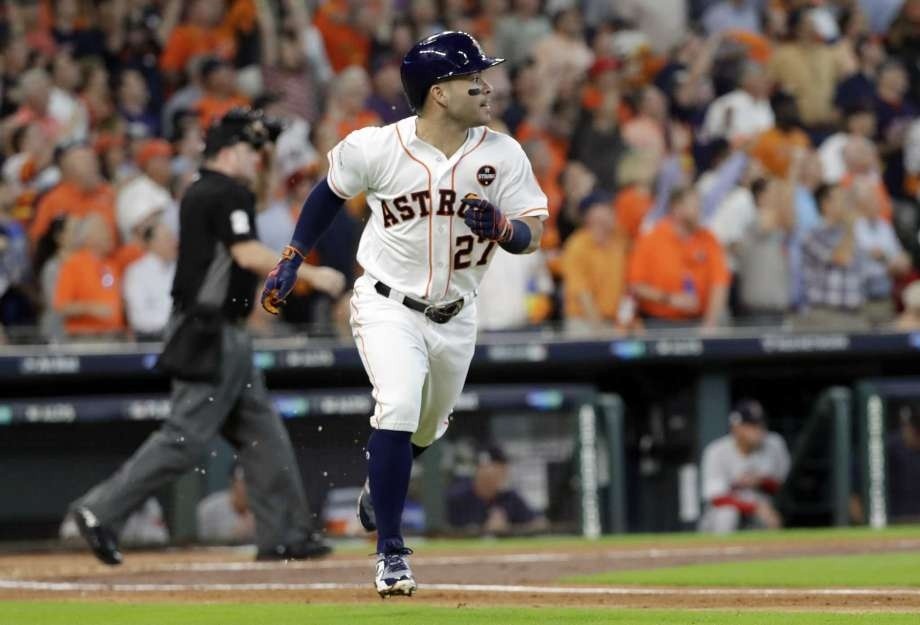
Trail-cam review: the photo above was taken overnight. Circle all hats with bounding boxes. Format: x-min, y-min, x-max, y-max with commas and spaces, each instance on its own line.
736, 401, 766, 422
137, 138, 176, 166
201, 120, 252, 152
580, 189, 614, 211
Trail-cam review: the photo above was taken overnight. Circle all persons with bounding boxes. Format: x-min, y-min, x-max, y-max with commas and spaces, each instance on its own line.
258, 31, 549, 595
346, 465, 426, 540
848, 404, 920, 525
0, 0, 920, 338
66, 101, 345, 566
696, 401, 792, 533
442, 447, 551, 539
62, 464, 254, 548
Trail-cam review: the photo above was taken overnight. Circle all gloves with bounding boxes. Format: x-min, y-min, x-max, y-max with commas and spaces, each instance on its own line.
260, 246, 307, 316
461, 196, 511, 244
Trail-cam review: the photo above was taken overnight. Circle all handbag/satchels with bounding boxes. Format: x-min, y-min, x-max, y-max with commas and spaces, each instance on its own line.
151, 312, 221, 387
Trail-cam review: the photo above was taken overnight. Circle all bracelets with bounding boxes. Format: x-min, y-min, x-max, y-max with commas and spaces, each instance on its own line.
660, 290, 672, 306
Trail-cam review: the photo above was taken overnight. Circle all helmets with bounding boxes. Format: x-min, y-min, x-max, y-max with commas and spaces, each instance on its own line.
401, 31, 505, 114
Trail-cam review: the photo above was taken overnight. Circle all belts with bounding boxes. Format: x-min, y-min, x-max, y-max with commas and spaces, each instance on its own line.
375, 280, 464, 325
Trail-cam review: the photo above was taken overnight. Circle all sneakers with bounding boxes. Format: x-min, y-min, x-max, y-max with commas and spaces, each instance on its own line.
357, 476, 377, 532
254, 539, 332, 563
368, 547, 418, 600
67, 498, 123, 566
